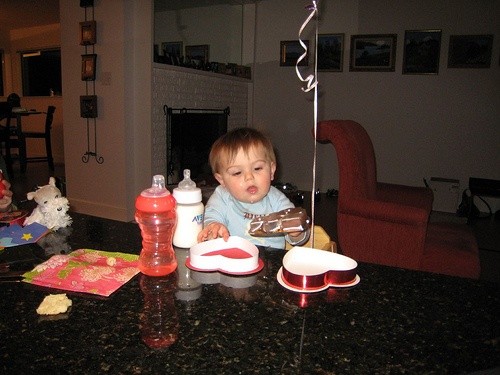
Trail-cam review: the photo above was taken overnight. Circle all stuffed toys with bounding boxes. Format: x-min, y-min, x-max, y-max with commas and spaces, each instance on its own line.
24, 176, 72, 230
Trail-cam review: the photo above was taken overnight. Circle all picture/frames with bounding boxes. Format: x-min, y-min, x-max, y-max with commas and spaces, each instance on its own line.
349, 33, 398, 72
160, 41, 183, 57
79, 95, 98, 118
314, 33, 345, 72
80, 54, 97, 81
401, 29, 442, 75
280, 40, 310, 67
447, 34, 494, 69
79, 20, 97, 46
185, 45, 210, 65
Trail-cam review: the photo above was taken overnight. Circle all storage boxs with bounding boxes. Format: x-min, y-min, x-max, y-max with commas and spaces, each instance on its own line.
429, 177, 460, 213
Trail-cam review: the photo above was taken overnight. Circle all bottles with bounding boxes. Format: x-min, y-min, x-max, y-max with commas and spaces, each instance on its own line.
171, 169, 205, 248
135, 175, 177, 276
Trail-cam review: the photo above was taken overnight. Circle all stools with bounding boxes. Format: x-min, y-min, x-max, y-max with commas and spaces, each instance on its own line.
467, 177, 500, 227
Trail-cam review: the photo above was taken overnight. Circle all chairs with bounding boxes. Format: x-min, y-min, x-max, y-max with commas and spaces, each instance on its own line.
16, 106, 56, 177
312, 119, 481, 279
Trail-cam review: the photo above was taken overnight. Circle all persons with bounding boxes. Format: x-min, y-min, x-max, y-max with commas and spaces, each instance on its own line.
0, 170, 17, 212
197, 127, 310, 250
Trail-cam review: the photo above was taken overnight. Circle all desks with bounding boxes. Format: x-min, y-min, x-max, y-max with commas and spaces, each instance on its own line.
0, 111, 48, 190
0, 211, 500, 375
